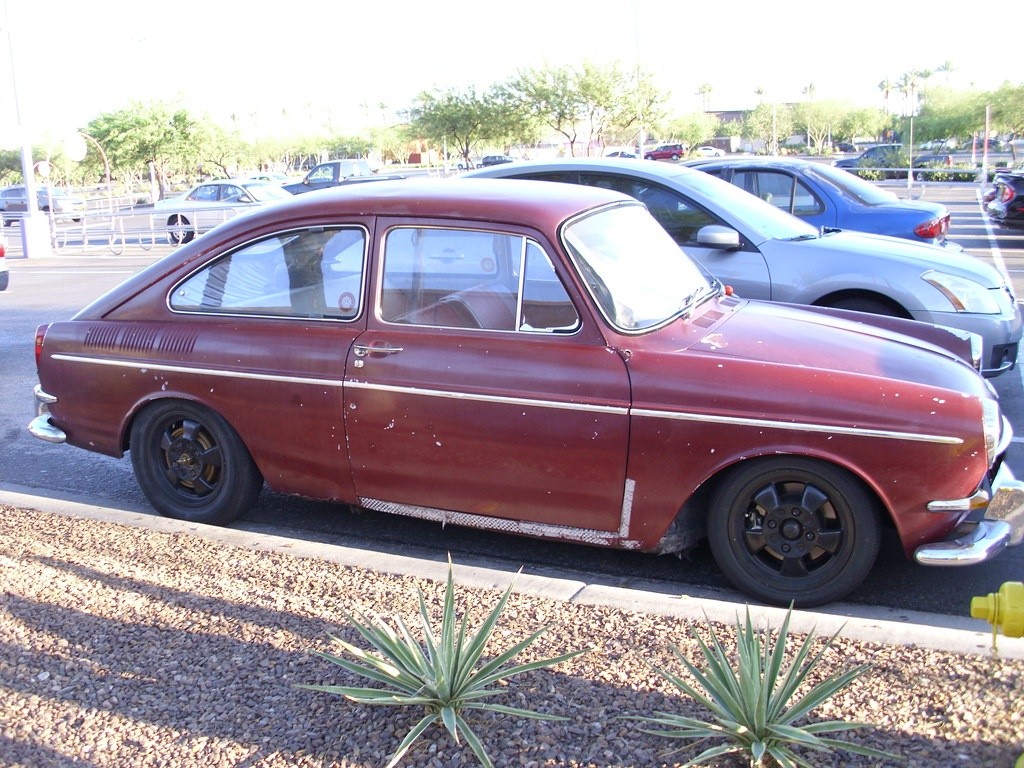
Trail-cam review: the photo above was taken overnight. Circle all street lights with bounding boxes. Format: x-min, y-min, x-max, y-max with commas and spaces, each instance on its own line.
64, 132, 114, 230
34, 161, 59, 250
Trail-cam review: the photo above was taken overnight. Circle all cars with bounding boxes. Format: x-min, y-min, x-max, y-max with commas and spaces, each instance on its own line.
482, 155, 514, 167
606, 151, 635, 158
27, 177, 1024, 608
693, 146, 726, 157
153, 178, 295, 243
460, 157, 1024, 380
831, 138, 1023, 230
689, 160, 964, 253
0, 186, 85, 226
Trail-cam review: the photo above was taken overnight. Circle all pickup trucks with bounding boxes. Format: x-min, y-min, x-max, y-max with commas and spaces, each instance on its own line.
281, 159, 407, 194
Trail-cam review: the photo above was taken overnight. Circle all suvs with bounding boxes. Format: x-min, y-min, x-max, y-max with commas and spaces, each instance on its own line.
644, 144, 684, 161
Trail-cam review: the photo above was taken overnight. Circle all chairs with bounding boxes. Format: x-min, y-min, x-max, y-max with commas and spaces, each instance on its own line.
391, 294, 485, 329
440, 281, 526, 332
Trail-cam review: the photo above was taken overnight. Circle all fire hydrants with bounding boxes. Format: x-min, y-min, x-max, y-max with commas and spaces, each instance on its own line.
970, 582, 1024, 768
